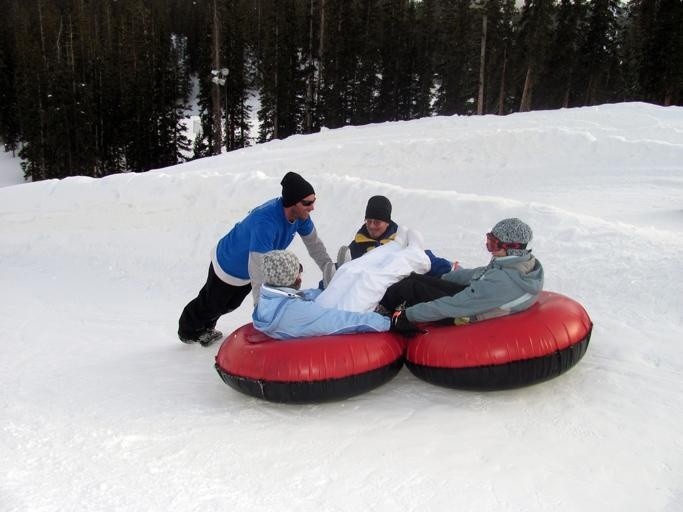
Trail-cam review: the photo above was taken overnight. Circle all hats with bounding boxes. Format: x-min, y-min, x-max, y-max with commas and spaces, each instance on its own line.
492, 218, 532, 244
365, 196, 391, 222
262, 250, 299, 286
281, 172, 314, 207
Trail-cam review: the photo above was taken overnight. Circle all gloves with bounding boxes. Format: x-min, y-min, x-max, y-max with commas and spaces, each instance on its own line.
425, 250, 451, 279
392, 310, 426, 338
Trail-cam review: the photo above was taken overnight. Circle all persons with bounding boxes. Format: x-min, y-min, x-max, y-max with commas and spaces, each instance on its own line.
253, 213, 440, 336
346, 196, 405, 257
323, 217, 545, 327
178, 171, 331, 343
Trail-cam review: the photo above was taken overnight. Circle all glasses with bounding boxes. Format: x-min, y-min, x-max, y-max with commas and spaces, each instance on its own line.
301, 200, 315, 206
486, 233, 526, 251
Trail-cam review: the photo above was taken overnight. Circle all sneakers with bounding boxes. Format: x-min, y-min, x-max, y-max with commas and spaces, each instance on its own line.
179, 329, 222, 346
